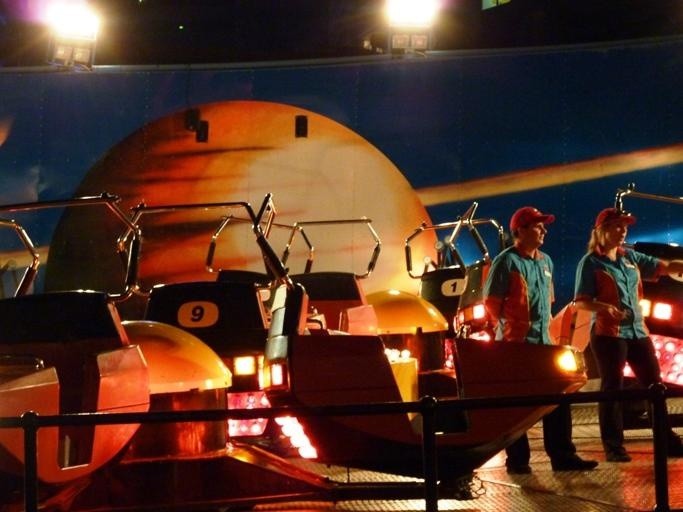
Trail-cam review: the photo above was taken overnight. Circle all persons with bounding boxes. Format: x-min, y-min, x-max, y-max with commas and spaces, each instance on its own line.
574, 207, 681, 462
483, 207, 598, 474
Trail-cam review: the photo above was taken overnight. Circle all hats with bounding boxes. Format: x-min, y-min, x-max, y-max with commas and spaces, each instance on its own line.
509, 205, 556, 232
593, 207, 637, 228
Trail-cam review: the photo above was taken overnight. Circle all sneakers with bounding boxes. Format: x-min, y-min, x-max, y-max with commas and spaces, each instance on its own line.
506, 462, 532, 474
666, 437, 682, 456
551, 455, 599, 471
605, 449, 631, 462
